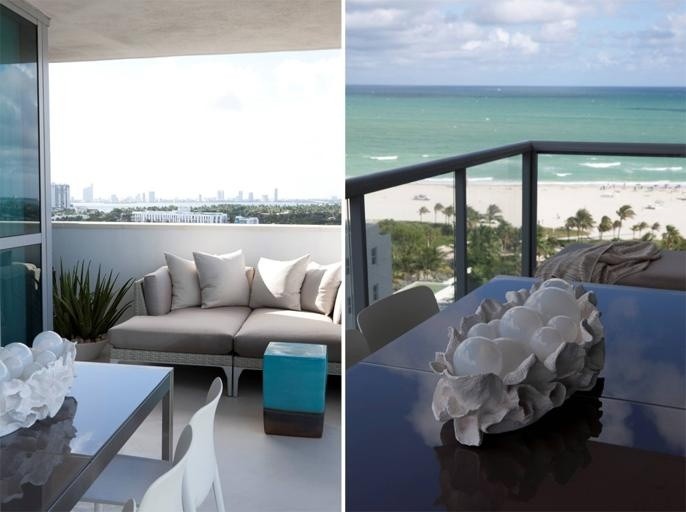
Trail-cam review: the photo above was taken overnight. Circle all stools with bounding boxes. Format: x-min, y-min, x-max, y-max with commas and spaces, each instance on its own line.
263, 340, 328, 437
539, 241, 685, 291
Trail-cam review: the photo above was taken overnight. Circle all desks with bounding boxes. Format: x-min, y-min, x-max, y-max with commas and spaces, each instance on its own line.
359, 272, 686, 410
345, 360, 686, 511
0, 361, 174, 512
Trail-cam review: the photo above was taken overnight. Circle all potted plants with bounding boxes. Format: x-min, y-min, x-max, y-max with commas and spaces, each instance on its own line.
52, 255, 136, 361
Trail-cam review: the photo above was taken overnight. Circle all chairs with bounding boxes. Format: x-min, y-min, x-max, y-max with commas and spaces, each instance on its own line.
345, 329, 371, 368
137, 425, 193, 512
79, 377, 224, 512
357, 284, 439, 355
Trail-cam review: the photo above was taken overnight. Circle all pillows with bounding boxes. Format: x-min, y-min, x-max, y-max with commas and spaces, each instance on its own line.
144, 250, 340, 315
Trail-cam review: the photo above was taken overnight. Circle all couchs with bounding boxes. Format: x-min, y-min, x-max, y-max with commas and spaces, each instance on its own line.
233, 277, 341, 398
107, 265, 253, 399
